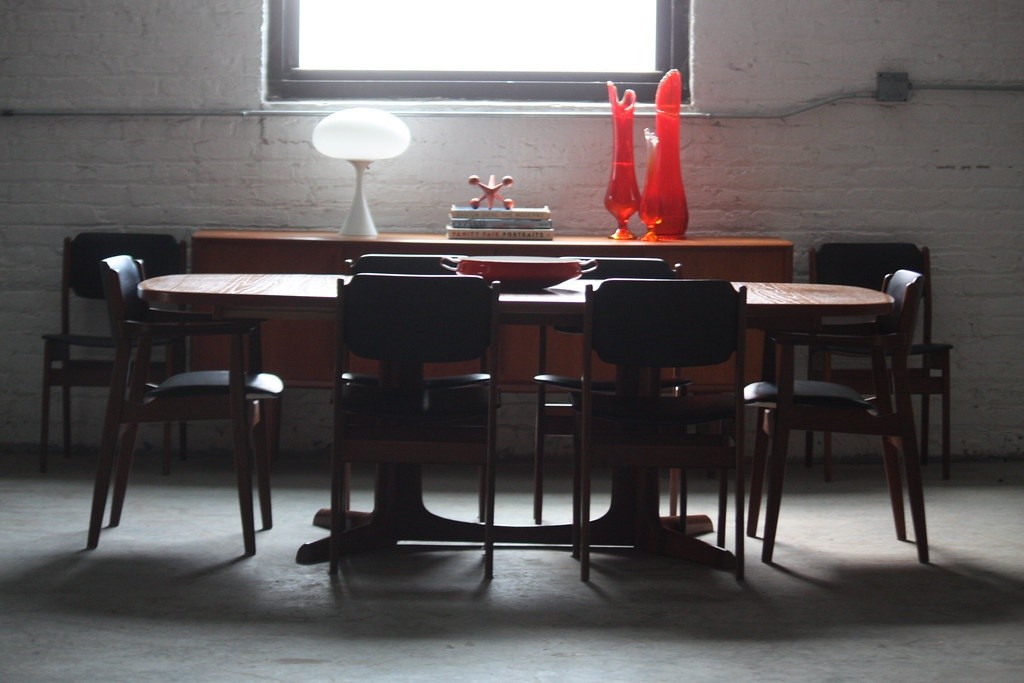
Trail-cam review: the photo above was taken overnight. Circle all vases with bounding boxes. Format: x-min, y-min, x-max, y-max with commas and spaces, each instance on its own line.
604, 80, 641, 240
638, 68, 690, 239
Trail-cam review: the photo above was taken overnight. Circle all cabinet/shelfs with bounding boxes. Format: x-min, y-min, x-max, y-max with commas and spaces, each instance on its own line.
189, 230, 792, 393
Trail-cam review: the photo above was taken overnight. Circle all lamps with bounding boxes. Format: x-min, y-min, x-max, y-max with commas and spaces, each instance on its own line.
311, 108, 409, 237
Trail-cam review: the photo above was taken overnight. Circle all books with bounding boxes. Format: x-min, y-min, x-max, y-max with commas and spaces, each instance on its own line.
444, 206, 555, 241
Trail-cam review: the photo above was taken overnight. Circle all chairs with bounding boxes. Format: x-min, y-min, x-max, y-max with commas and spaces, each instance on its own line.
574, 277, 747, 584
804, 241, 955, 479
42, 232, 192, 474
329, 275, 499, 581
336, 254, 498, 522
87, 254, 285, 556
533, 257, 687, 524
743, 271, 932, 563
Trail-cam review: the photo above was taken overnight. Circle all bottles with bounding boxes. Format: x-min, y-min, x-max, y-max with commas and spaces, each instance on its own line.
655, 69, 690, 240
638, 128, 667, 243
604, 81, 641, 240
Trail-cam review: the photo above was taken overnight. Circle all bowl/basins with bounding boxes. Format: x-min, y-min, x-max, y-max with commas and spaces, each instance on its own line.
439, 256, 598, 289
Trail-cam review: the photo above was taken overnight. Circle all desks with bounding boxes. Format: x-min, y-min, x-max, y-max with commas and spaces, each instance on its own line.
135, 274, 894, 542
